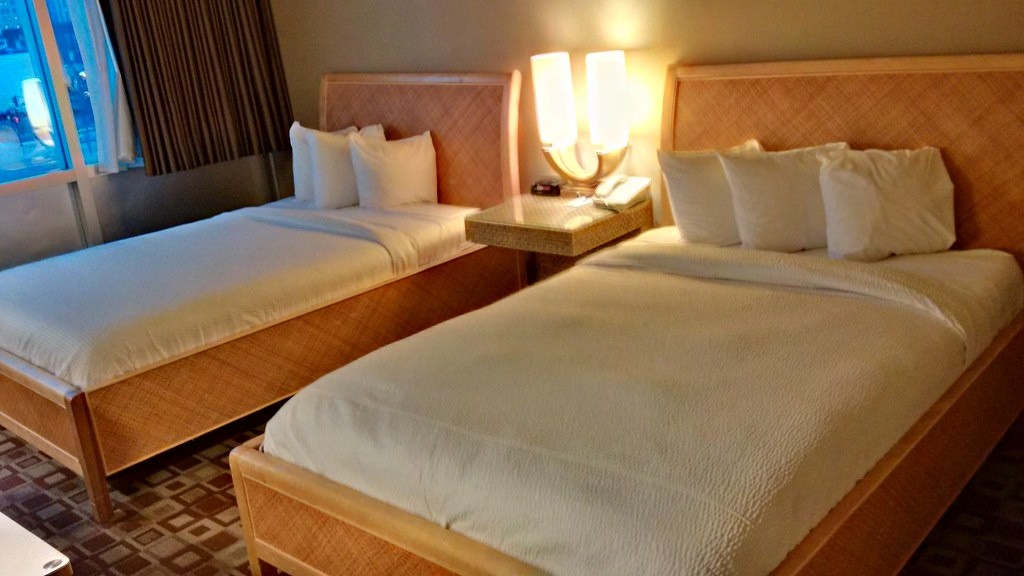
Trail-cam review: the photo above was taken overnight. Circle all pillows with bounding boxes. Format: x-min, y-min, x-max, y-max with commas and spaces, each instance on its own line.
349, 129, 437, 210
304, 123, 387, 210
656, 139, 766, 247
815, 146, 957, 261
714, 142, 851, 253
289, 121, 359, 205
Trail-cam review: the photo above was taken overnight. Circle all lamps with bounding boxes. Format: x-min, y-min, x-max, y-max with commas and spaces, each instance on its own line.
530, 50, 628, 199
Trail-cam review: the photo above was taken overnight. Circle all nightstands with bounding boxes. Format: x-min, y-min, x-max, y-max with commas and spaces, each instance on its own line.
465, 189, 657, 290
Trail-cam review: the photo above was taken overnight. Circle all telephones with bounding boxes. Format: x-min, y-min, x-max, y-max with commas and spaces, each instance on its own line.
592, 172, 653, 212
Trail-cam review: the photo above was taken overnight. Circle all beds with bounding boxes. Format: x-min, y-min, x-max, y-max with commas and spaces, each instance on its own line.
0, 69, 530, 526
230, 53, 1024, 576
